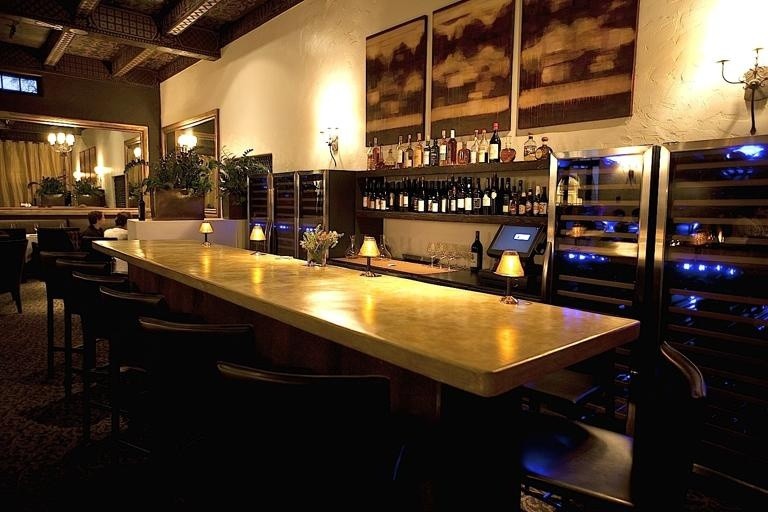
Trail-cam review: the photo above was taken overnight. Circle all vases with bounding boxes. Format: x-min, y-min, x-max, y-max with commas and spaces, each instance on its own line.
307, 250, 327, 264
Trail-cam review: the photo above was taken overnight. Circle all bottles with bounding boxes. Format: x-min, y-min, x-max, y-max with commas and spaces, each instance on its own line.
478, 129, 488, 163
372, 138, 379, 163
509, 185, 517, 215
517, 180, 522, 199
457, 140, 470, 164
523, 132, 537, 161
489, 123, 501, 163
361, 176, 464, 213
430, 139, 438, 166
447, 129, 457, 165
500, 142, 516, 162
525, 184, 533, 217
375, 146, 385, 170
367, 142, 375, 171
470, 130, 480, 163
403, 134, 413, 168
518, 190, 526, 215
422, 136, 430, 167
502, 178, 511, 215
533, 185, 540, 217
472, 177, 483, 215
490, 173, 500, 215
384, 149, 395, 169
535, 137, 553, 160
438, 129, 447, 166
482, 177, 491, 215
395, 136, 404, 168
470, 231, 483, 270
464, 177, 473, 215
413, 133, 424, 167
539, 187, 548, 217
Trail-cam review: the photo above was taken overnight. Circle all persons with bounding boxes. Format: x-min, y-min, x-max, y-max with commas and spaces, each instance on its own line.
81, 211, 105, 237
104, 212, 132, 240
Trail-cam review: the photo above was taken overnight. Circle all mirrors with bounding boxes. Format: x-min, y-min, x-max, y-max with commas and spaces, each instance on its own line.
159, 110, 221, 219
0, 112, 152, 217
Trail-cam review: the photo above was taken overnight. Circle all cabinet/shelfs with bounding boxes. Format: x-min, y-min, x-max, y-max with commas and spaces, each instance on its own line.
354, 154, 551, 226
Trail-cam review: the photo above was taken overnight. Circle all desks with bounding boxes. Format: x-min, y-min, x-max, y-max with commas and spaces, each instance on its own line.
91, 236, 639, 433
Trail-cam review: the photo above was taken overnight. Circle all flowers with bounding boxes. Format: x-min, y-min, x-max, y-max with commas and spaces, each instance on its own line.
298, 224, 344, 260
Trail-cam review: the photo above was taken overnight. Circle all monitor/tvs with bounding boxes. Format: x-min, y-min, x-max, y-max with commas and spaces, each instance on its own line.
486, 222, 545, 260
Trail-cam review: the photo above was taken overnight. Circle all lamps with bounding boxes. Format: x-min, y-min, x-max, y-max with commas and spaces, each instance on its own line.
45, 131, 75, 159
196, 222, 214, 246
319, 122, 340, 169
248, 224, 269, 255
359, 236, 384, 277
704, 15, 768, 136
494, 251, 527, 305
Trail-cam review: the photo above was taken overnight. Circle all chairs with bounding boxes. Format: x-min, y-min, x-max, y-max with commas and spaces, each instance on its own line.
97, 287, 182, 458
509, 340, 715, 512
29, 244, 102, 377
44, 255, 113, 412
1, 227, 28, 318
31, 223, 81, 259
81, 236, 121, 260
61, 270, 131, 453
515, 260, 652, 433
213, 353, 427, 512
123, 313, 258, 470
